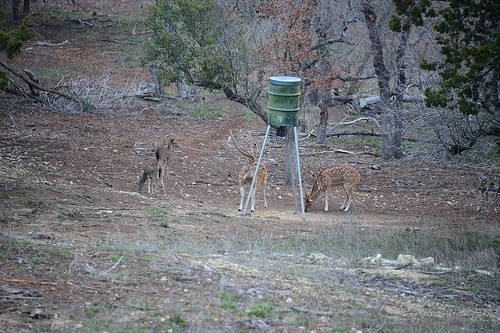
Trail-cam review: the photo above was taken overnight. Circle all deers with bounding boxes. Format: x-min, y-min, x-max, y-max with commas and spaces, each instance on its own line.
304, 164, 361, 213
229, 128, 269, 214
155, 137, 179, 179
139, 161, 166, 199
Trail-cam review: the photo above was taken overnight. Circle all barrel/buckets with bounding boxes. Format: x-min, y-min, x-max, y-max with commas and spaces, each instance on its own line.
268, 73, 302, 126
